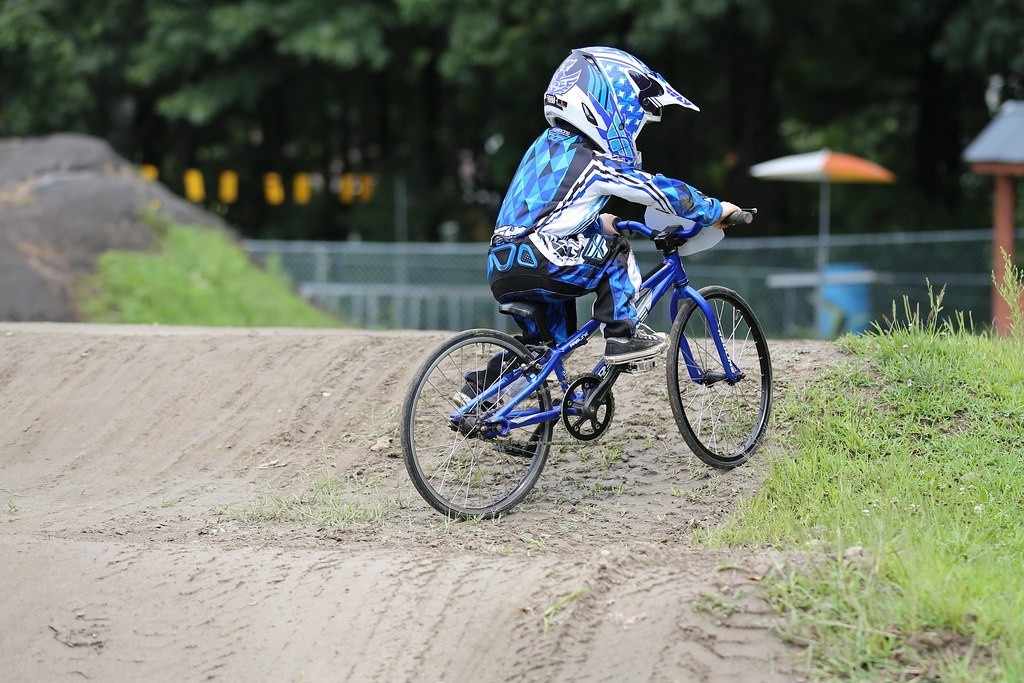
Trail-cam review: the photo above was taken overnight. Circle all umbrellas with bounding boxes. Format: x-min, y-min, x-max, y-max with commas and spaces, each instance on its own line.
749, 145, 897, 271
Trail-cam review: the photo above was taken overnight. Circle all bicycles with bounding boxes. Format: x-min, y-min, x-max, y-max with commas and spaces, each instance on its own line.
398, 206, 774, 525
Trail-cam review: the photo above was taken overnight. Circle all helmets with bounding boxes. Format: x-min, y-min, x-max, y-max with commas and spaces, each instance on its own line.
543, 46, 701, 170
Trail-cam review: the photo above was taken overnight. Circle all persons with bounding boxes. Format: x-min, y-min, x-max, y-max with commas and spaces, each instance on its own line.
454, 46, 742, 416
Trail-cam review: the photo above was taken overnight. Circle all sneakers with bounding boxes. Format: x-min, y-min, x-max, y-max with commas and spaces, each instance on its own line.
602, 322, 671, 365
453, 382, 496, 415
